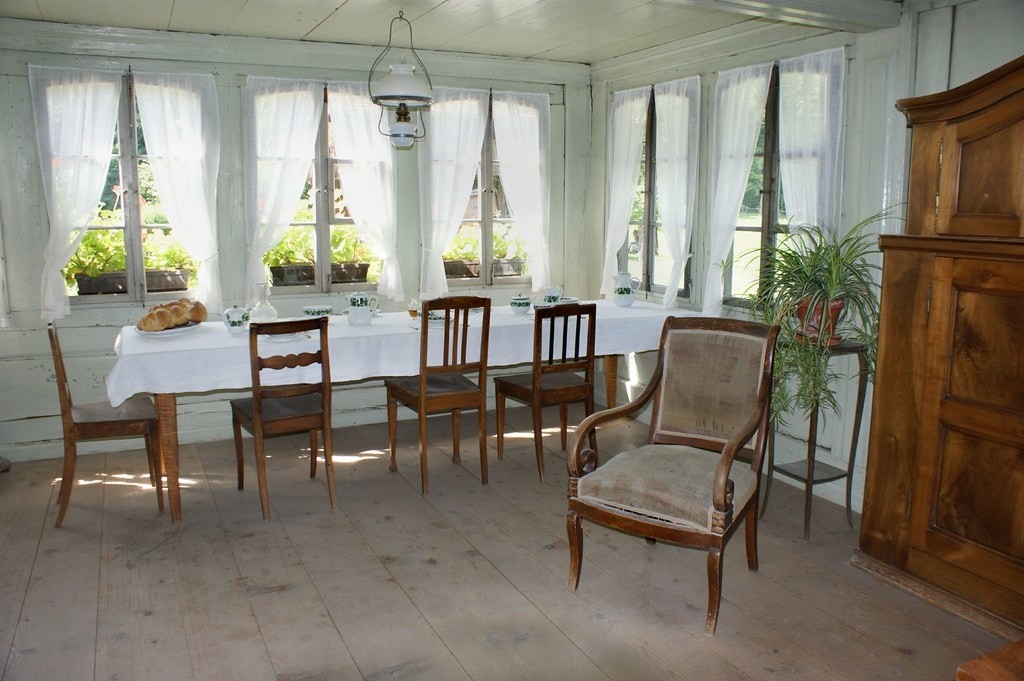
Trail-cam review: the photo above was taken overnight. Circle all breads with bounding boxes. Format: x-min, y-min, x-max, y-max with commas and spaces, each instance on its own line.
136, 298, 208, 331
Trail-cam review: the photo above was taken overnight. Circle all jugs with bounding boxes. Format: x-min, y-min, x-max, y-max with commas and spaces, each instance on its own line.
219, 305, 253, 337
612, 272, 640, 307
345, 289, 379, 326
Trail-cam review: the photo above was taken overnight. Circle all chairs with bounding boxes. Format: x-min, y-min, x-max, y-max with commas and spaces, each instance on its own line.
493, 303, 598, 483
230, 316, 338, 523
565, 315, 781, 637
47, 320, 165, 528
384, 296, 491, 496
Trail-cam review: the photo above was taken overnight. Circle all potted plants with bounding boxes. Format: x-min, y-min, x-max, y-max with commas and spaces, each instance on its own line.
60, 228, 191, 295
442, 233, 526, 278
262, 231, 370, 286
713, 201, 907, 432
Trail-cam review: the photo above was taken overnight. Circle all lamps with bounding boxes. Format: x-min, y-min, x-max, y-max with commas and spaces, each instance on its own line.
368, 10, 434, 151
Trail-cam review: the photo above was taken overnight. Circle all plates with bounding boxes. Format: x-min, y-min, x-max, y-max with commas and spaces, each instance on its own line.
135, 322, 201, 337
343, 310, 382, 317
419, 312, 454, 323
534, 306, 553, 311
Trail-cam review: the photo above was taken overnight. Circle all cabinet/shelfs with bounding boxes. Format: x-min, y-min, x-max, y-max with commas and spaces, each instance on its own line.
847, 58, 1024, 643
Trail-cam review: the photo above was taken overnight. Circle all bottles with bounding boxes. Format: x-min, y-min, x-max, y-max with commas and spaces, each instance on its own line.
250, 284, 277, 323
409, 298, 417, 320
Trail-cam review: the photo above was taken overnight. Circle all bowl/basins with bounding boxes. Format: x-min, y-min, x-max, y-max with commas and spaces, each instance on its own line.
303, 306, 332, 319
561, 298, 577, 307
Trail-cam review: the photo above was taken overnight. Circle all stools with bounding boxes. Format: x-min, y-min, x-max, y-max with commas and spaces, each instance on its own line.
759, 340, 869, 542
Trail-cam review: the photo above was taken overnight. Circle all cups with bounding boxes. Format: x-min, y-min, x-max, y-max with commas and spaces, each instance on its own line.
510, 293, 535, 315
544, 287, 562, 305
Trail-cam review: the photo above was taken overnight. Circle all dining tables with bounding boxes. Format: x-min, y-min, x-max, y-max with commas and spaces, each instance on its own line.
104, 299, 721, 524
